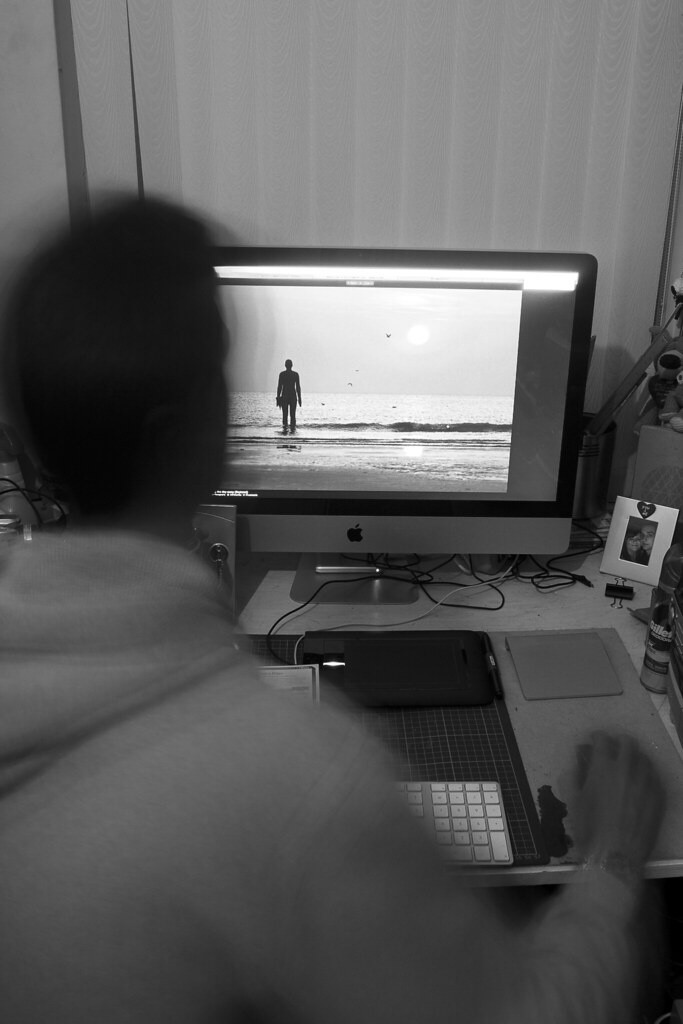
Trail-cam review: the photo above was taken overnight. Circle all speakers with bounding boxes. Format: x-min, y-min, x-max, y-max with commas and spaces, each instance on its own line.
629, 425, 683, 531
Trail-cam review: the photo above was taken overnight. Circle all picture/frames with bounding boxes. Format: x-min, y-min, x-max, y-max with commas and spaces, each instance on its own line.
599, 495, 680, 590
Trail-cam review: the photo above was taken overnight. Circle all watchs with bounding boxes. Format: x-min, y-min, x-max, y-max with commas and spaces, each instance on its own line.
578, 849, 648, 899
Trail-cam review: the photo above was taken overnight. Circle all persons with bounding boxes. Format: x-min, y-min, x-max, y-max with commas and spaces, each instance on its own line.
620, 523, 656, 566
0, 195, 661, 1023
276, 359, 302, 436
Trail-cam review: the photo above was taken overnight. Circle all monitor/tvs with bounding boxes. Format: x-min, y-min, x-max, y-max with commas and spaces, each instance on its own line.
208, 247, 599, 607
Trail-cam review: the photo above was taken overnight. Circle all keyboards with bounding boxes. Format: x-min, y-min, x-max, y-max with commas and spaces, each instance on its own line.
392, 780, 514, 867
